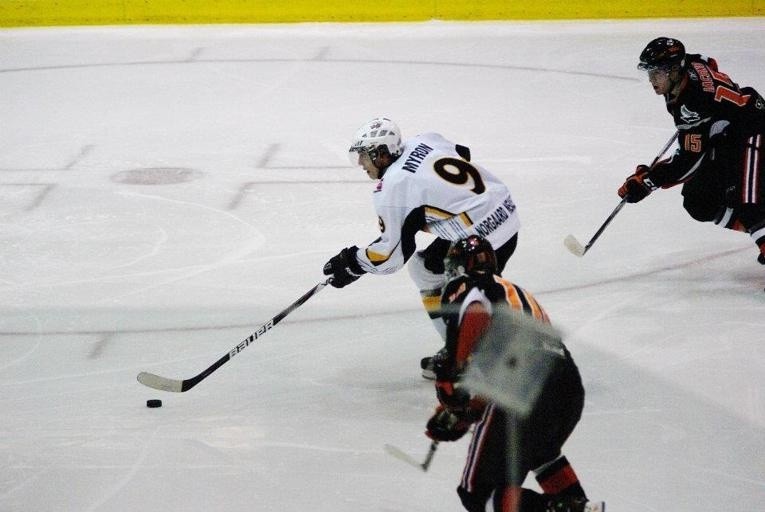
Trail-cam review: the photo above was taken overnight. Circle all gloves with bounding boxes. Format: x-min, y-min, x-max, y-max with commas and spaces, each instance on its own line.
617, 164, 657, 203
423, 374, 484, 443
324, 245, 368, 290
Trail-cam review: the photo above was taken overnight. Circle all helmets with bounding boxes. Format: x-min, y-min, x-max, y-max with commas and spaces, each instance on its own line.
637, 38, 685, 72
347, 117, 402, 158
442, 234, 500, 280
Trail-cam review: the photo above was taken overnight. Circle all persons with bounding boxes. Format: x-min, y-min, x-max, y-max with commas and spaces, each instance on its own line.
424, 231, 607, 512
321, 116, 523, 374
616, 37, 765, 270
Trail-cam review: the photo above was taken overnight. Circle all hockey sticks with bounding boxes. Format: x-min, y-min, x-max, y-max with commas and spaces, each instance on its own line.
137, 277, 336, 391
384, 441, 437, 472
563, 131, 679, 258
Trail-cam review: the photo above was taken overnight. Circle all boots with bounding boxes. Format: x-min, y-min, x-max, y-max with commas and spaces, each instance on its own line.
419, 349, 449, 370
541, 496, 591, 512
